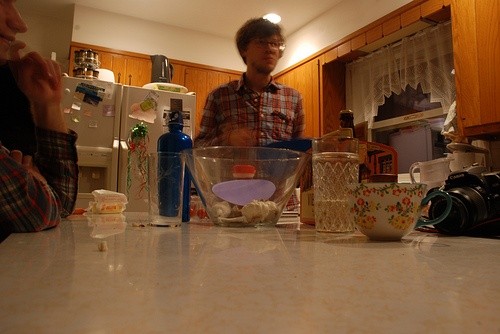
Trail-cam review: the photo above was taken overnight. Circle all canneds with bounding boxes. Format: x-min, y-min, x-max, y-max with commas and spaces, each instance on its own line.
73, 49, 99, 80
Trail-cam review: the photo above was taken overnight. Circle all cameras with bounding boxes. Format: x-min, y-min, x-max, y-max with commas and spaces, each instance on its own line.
427, 171, 500, 236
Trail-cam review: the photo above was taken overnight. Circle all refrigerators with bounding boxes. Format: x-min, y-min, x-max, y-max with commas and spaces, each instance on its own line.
60, 74, 197, 213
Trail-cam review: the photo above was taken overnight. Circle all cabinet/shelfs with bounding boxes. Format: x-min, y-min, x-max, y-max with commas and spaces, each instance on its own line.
453, 0, 500, 127
69, 44, 319, 139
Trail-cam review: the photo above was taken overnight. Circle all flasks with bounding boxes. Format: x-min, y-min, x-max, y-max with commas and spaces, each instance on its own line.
157, 111, 193, 224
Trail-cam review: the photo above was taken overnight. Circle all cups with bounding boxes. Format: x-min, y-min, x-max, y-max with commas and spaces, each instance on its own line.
311, 136, 359, 235
449, 152, 485, 172
344, 182, 453, 242
147, 151, 185, 229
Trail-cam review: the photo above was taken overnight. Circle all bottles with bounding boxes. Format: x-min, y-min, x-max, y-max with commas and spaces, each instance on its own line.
337, 109, 368, 183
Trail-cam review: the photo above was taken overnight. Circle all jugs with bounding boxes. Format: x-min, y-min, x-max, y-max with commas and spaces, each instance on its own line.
150, 55, 174, 83
409, 157, 455, 190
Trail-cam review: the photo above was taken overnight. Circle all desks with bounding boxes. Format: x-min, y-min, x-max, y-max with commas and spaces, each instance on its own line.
1, 211, 500, 334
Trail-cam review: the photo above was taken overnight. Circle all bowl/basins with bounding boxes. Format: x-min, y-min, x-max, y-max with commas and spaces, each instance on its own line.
180, 146, 312, 229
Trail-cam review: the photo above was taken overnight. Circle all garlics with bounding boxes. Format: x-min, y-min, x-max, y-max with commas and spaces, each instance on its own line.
210, 200, 277, 223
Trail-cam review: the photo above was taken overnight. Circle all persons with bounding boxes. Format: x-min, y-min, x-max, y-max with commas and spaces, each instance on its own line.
0, 0, 79, 245
193, 18, 305, 211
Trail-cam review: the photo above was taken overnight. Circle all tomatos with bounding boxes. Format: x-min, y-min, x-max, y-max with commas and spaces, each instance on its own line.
188, 207, 207, 218
